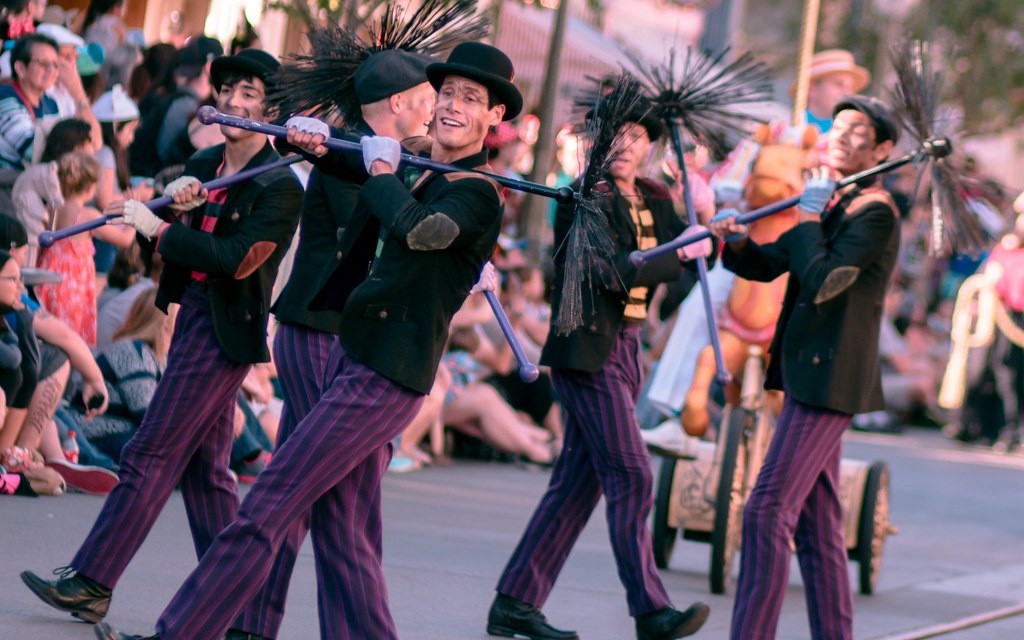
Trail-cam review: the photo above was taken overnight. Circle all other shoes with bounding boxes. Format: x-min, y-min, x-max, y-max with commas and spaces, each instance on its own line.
532, 427, 559, 445
44, 458, 120, 495
385, 449, 421, 472
518, 452, 558, 469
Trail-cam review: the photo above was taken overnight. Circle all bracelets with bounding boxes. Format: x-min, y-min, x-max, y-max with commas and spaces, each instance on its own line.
74, 98, 91, 110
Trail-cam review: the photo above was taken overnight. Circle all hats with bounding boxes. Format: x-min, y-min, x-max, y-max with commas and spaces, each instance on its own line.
34, 23, 86, 47
425, 41, 523, 122
354, 50, 445, 104
832, 94, 901, 142
0, 216, 28, 273
788, 49, 871, 93
175, 36, 224, 78
91, 83, 141, 121
210, 49, 291, 125
585, 90, 664, 142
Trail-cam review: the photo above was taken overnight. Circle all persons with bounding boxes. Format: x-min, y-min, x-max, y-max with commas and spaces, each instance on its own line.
485, 89, 717, 640
226, 50, 436, 640
0, 1, 1024, 498
640, 47, 871, 460
20, 47, 305, 625
95, 40, 523, 640
709, 94, 901, 640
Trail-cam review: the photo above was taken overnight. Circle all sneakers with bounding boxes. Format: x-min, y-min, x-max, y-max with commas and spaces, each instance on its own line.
239, 449, 276, 484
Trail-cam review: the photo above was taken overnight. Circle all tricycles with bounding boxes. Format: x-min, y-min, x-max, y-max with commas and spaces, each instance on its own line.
652, 2, 899, 593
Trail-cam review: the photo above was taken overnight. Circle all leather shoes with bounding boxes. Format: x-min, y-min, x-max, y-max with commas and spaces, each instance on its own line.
635, 602, 710, 640
224, 629, 273, 640
93, 623, 160, 640
486, 592, 580, 640
20, 570, 112, 623
638, 420, 700, 461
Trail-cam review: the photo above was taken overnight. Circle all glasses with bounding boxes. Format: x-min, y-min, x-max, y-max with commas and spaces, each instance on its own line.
60, 50, 80, 61
0, 276, 24, 284
31, 58, 59, 68
